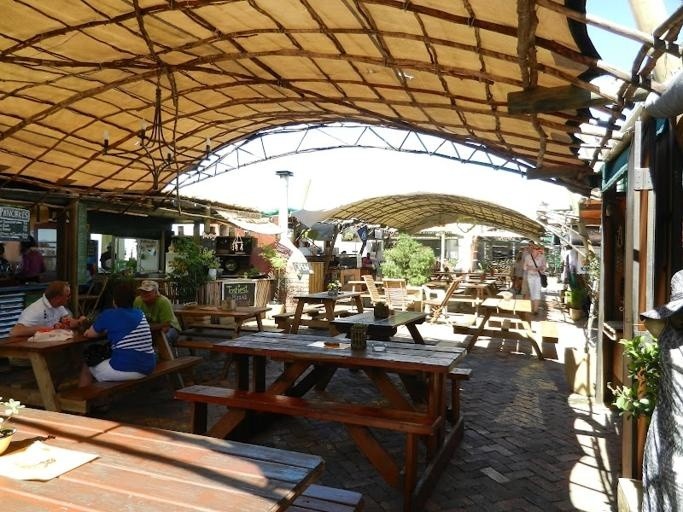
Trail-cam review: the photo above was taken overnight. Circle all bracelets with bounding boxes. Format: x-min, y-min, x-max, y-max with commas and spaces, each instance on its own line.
77, 319, 80, 326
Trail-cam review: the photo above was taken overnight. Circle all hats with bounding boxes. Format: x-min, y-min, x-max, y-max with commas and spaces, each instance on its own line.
134, 280, 159, 293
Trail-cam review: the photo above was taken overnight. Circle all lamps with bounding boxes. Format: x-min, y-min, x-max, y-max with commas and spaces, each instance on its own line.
100, 75, 214, 191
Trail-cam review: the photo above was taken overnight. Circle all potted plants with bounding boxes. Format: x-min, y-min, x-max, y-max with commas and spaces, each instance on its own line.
565, 286, 589, 322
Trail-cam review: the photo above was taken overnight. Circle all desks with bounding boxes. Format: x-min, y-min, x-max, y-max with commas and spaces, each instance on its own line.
0, 263, 561, 511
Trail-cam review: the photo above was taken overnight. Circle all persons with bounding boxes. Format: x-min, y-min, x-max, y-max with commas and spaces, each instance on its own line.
75, 283, 157, 413
522, 244, 546, 316
7, 280, 90, 382
521, 241, 535, 300
16, 234, 48, 276
131, 279, 183, 359
0, 242, 14, 276
513, 248, 528, 295
361, 253, 372, 267
638, 270, 683, 511
98, 242, 117, 271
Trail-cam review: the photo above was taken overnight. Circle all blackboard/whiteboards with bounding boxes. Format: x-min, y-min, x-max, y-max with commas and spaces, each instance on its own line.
0, 202, 32, 243
222, 282, 257, 307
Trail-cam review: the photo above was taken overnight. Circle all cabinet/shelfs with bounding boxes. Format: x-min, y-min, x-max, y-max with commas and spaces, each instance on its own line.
212, 236, 258, 277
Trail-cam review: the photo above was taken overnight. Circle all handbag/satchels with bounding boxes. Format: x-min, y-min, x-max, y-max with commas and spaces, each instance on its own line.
539, 275, 548, 288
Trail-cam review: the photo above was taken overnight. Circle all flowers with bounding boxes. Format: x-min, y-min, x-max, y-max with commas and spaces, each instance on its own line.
603, 331, 660, 416
